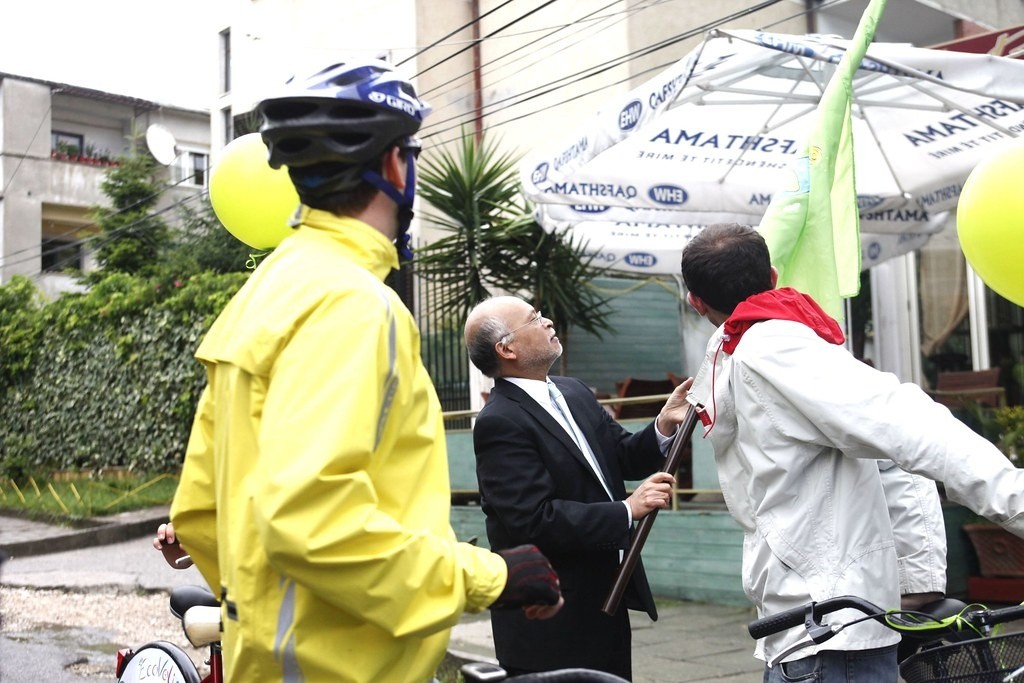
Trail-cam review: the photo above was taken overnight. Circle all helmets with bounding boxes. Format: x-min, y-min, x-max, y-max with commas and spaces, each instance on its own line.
256, 46, 432, 169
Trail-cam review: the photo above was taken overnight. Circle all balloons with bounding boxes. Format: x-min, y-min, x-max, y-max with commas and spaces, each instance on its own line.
208, 131, 302, 253
955, 146, 1024, 309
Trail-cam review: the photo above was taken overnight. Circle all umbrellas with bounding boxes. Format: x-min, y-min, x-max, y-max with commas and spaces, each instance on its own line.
522, 27, 1024, 283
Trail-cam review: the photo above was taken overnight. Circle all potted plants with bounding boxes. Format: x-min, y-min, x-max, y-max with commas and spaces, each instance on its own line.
51, 140, 130, 167
960, 393, 1023, 578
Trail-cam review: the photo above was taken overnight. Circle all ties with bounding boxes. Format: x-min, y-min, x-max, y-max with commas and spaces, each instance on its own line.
548, 384, 607, 488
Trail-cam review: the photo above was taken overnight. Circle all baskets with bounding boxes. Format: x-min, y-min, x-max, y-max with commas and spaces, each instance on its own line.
900, 632, 1024, 683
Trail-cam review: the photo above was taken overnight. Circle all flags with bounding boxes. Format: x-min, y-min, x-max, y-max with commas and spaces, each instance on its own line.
757, 0, 885, 318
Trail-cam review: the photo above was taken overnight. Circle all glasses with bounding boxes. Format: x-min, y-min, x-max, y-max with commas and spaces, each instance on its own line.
392, 140, 421, 160
500, 311, 542, 342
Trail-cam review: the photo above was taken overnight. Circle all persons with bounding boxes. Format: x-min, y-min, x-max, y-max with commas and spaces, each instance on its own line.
463, 296, 694, 683
681, 224, 1024, 683
875, 458, 947, 666
151, 51, 563, 682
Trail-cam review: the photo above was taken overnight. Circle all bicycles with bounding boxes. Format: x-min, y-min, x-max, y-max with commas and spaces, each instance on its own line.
114, 532, 629, 683
745, 595, 1023, 683
